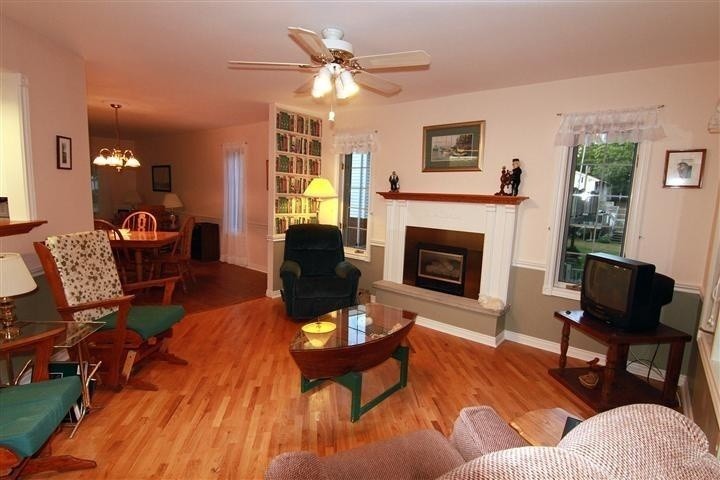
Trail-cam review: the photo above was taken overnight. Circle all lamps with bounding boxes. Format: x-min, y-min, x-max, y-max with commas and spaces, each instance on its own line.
302, 178, 339, 225
310, 63, 359, 123
0, 252, 38, 344
94, 104, 142, 173
162, 193, 184, 232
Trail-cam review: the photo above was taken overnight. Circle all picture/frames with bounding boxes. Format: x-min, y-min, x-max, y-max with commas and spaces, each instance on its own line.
152, 165, 172, 192
422, 121, 485, 172
662, 149, 706, 188
56, 135, 72, 170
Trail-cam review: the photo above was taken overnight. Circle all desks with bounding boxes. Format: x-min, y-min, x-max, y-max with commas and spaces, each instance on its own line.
547, 308, 691, 418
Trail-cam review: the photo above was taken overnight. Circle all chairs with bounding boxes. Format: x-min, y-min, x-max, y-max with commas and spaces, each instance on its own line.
32, 228, 189, 392
278, 224, 362, 325
263, 406, 720, 480
0, 375, 97, 480
93, 204, 199, 296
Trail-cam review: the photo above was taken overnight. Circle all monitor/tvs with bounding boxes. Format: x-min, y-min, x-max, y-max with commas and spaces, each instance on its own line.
581, 252, 674, 333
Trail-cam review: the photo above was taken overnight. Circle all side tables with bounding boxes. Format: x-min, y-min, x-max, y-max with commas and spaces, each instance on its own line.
14, 322, 109, 412
509, 408, 584, 447
0, 321, 67, 383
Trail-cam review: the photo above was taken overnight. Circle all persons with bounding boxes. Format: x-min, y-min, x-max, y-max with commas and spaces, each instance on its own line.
509, 157, 523, 196
388, 171, 400, 193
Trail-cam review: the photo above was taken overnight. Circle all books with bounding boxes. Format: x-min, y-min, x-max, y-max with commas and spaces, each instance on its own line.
49, 362, 96, 424
276, 109, 324, 233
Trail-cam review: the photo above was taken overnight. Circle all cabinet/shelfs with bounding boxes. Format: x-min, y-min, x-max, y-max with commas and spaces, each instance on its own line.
190, 222, 220, 263
267, 105, 323, 299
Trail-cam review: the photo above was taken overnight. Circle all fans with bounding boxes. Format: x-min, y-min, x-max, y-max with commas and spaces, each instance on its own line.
227, 26, 431, 123
